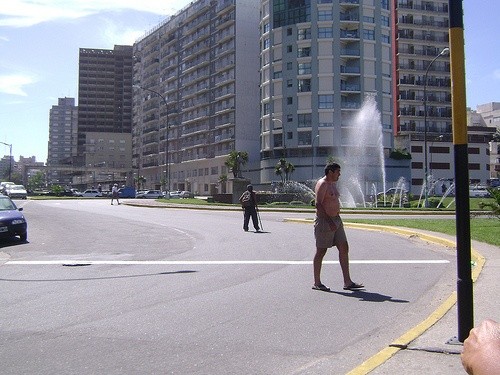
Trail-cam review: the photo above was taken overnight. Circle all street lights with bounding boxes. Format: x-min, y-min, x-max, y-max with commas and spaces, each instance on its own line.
424, 48, 450, 208
272, 118, 285, 160
430, 134, 443, 194
132, 85, 168, 179
311, 134, 320, 179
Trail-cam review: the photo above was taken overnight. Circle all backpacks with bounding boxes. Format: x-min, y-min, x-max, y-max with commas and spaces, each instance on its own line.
241, 191, 253, 208
113, 190, 116, 198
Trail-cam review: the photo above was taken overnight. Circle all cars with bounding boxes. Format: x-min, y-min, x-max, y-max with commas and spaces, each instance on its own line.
33, 188, 124, 197
135, 188, 195, 199
0, 182, 28, 241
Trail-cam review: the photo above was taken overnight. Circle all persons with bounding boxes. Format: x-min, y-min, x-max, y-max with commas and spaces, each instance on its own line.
111, 184, 121, 205
239, 184, 260, 232
312, 163, 365, 291
461, 319, 500, 375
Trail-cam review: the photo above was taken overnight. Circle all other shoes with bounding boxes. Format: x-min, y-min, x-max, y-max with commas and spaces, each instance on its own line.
342, 282, 365, 290
312, 281, 331, 291
